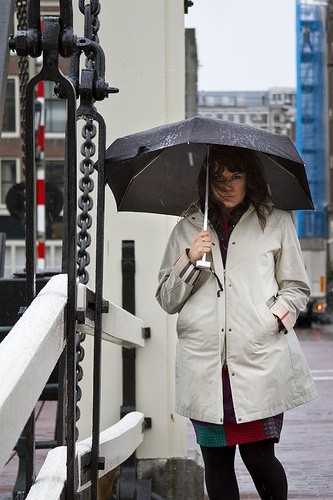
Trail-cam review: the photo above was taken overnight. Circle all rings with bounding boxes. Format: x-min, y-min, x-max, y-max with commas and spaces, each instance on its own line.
202, 236, 205, 242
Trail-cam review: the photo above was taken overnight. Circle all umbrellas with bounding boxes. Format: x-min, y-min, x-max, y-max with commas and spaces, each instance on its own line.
91, 116, 316, 297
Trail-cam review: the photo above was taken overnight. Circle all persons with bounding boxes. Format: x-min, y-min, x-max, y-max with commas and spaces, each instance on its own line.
156, 143, 318, 500
